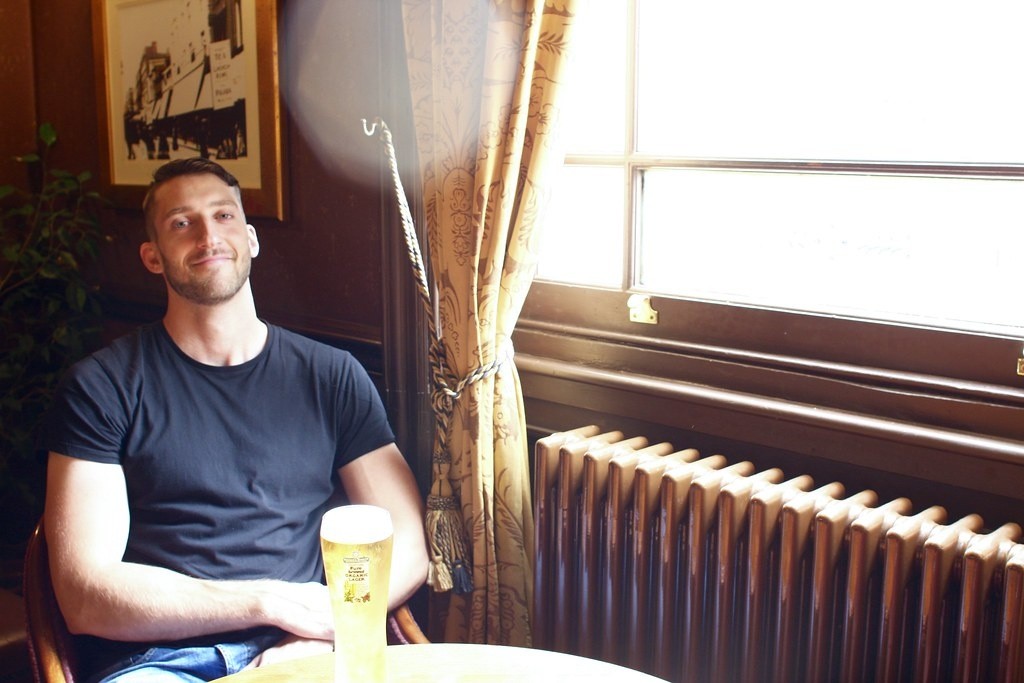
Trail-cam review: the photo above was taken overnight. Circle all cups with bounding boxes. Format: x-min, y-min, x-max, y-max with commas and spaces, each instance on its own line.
321, 504, 393, 683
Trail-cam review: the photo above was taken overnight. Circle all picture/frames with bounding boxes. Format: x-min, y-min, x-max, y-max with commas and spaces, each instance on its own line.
91, 0, 291, 223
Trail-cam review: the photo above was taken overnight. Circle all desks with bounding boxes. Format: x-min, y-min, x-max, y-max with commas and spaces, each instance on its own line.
203, 643, 671, 683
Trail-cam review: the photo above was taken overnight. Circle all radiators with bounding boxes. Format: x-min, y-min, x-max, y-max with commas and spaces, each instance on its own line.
524, 423, 1024, 683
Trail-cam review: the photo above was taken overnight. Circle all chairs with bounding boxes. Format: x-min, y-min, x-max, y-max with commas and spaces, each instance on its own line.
19, 513, 434, 683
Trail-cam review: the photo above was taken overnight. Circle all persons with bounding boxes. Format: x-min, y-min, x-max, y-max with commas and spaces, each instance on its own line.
43, 158, 432, 683
